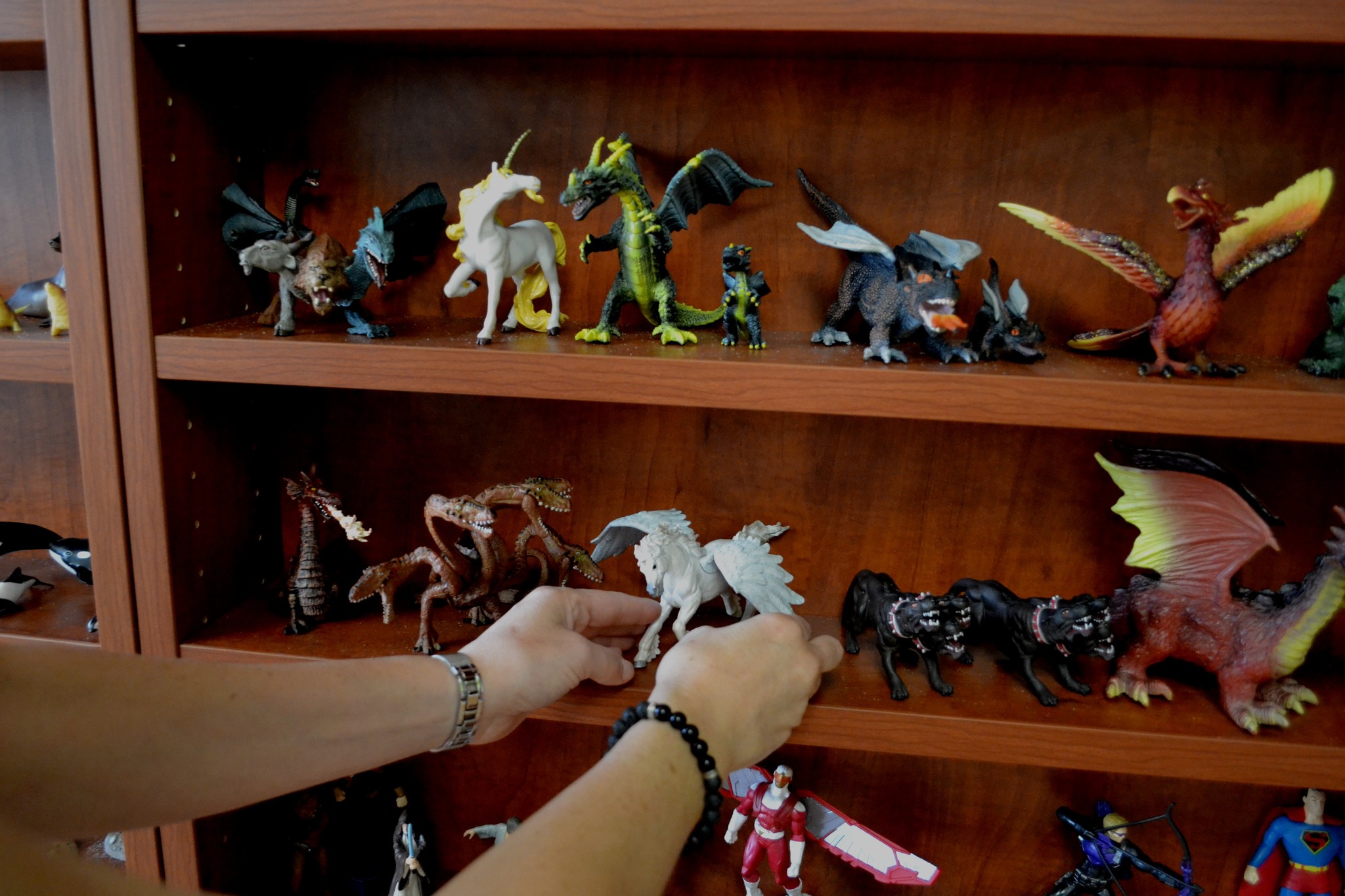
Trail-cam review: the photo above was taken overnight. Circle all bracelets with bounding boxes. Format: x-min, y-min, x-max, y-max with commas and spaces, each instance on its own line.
431, 651, 485, 754
604, 699, 724, 858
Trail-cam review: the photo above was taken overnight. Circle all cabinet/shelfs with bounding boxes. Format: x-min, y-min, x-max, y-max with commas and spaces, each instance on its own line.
0, 0, 1345, 896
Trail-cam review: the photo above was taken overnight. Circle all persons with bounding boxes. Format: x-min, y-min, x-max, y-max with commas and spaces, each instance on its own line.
0, 583, 846, 896
725, 765, 808, 896
389, 809, 427, 896
1048, 801, 1199, 896
1239, 787, 1345, 896
